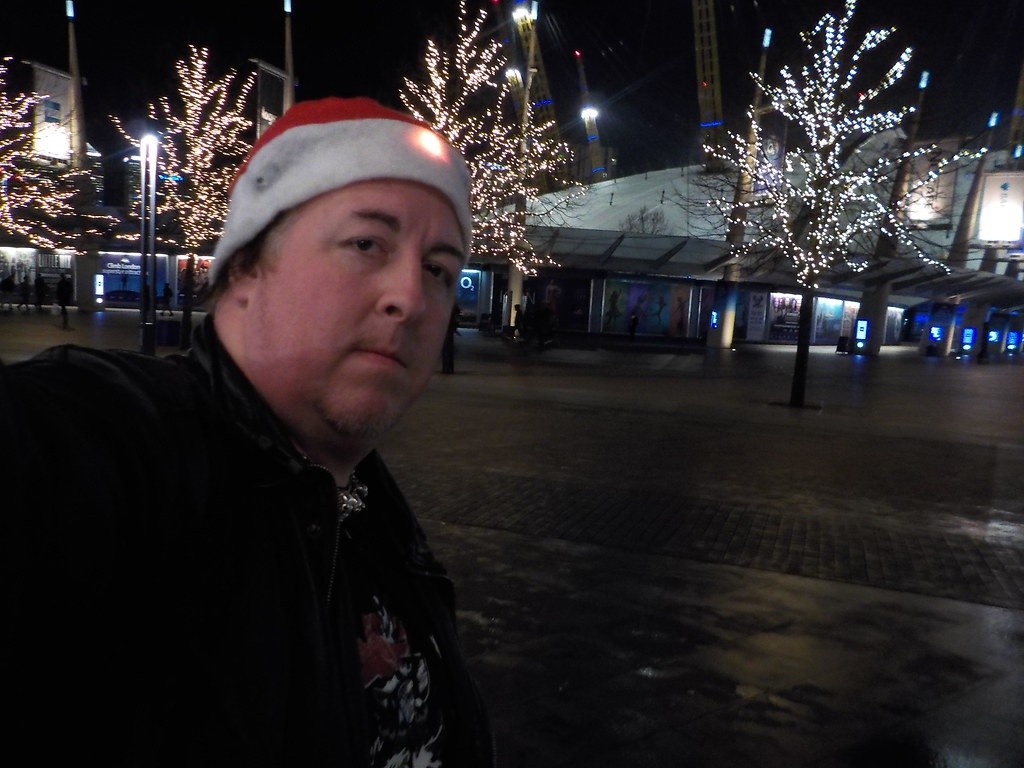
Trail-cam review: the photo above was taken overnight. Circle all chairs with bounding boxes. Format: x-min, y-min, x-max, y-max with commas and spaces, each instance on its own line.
479, 313, 489, 331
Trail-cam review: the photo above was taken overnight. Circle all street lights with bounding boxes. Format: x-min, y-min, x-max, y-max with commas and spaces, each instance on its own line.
138, 133, 159, 354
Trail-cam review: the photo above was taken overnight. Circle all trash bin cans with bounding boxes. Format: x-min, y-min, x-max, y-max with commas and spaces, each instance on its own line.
836, 336, 850, 352
157, 320, 181, 347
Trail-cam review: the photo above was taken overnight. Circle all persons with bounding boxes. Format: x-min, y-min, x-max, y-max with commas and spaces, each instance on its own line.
160, 282, 174, 317
0, 273, 52, 313
545, 279, 563, 300
628, 312, 638, 340
55, 273, 72, 325
772, 298, 797, 313
515, 304, 523, 339
0, 98, 496, 768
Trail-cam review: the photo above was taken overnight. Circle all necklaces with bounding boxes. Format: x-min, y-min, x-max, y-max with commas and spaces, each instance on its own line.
337, 470, 370, 515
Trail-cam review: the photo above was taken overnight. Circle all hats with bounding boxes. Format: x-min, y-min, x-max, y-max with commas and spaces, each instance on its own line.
210, 97, 473, 287
